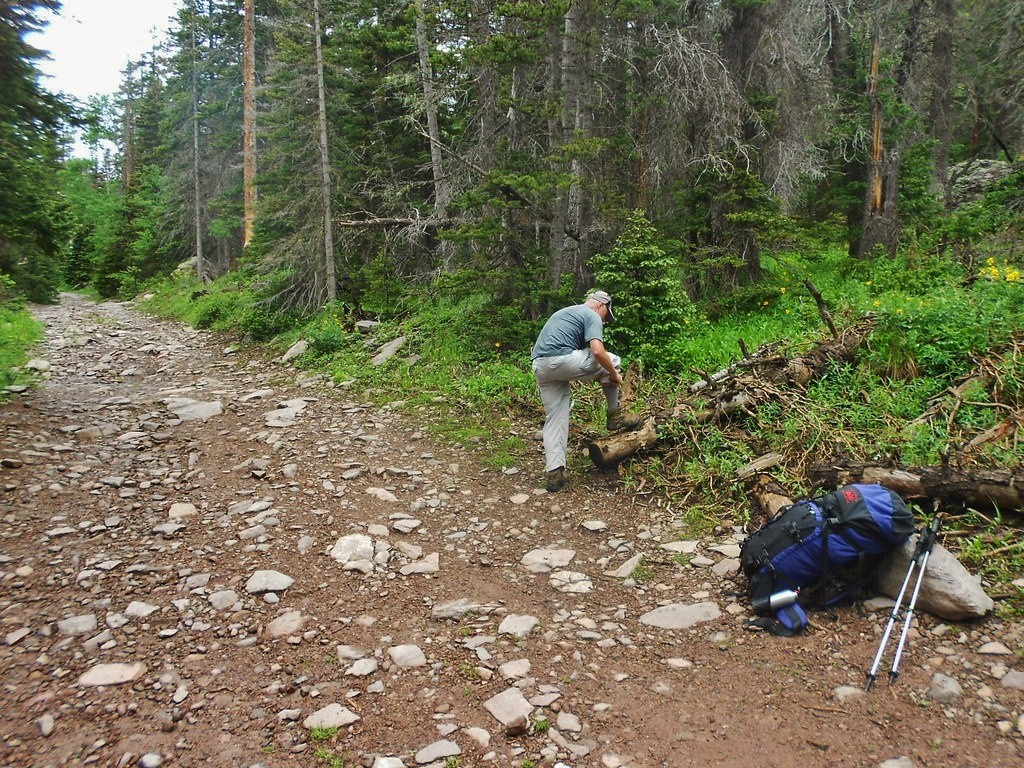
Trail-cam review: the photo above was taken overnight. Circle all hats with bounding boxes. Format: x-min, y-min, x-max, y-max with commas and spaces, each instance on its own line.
588, 291, 614, 323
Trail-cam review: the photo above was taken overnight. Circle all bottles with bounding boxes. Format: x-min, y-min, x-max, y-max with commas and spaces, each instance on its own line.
770, 587, 800, 611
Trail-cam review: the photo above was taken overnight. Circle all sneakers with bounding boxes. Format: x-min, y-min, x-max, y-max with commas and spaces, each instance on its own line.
546, 467, 570, 492
606, 406, 639, 431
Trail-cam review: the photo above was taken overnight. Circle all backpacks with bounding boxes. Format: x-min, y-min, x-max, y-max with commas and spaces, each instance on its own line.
720, 483, 915, 637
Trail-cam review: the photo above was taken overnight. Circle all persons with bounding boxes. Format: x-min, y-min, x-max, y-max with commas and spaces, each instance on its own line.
532, 292, 640, 492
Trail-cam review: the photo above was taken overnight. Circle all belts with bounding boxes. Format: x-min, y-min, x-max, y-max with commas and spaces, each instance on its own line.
538, 355, 549, 357
